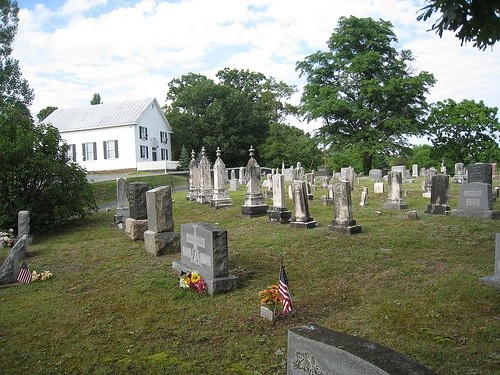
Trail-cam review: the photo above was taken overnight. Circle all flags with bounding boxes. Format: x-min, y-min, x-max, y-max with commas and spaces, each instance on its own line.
17, 261, 32, 284
278, 265, 293, 314
